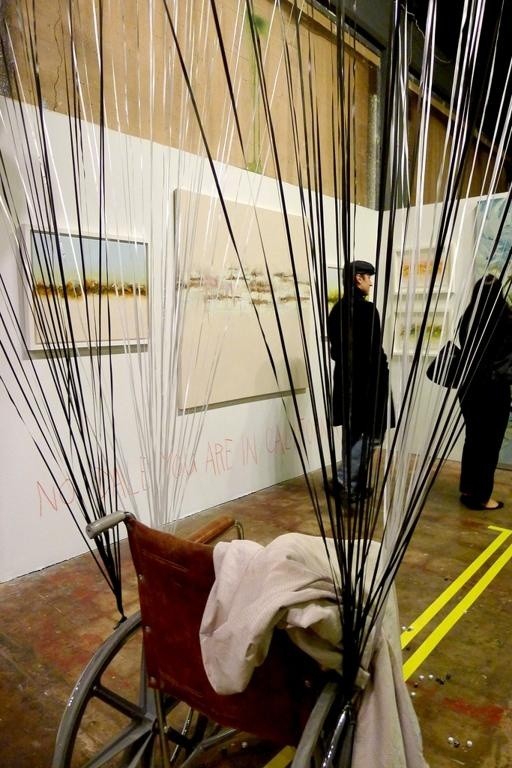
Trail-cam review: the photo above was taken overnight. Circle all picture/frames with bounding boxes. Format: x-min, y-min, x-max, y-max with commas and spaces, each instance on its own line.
16, 223, 150, 353
390, 240, 456, 295
326, 265, 373, 317
387, 307, 453, 357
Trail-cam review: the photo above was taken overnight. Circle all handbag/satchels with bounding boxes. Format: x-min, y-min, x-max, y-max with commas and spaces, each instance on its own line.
422, 332, 468, 389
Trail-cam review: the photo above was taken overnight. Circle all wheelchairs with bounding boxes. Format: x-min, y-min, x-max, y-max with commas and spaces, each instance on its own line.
47, 509, 419, 766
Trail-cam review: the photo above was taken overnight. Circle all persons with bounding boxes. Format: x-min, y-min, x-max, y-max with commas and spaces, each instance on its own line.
456, 273, 512, 510
324, 260, 397, 501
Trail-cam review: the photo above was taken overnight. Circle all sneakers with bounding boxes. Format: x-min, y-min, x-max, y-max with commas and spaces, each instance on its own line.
322, 478, 375, 517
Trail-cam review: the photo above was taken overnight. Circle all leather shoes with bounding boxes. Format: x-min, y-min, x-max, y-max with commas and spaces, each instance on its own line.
459, 493, 504, 511
